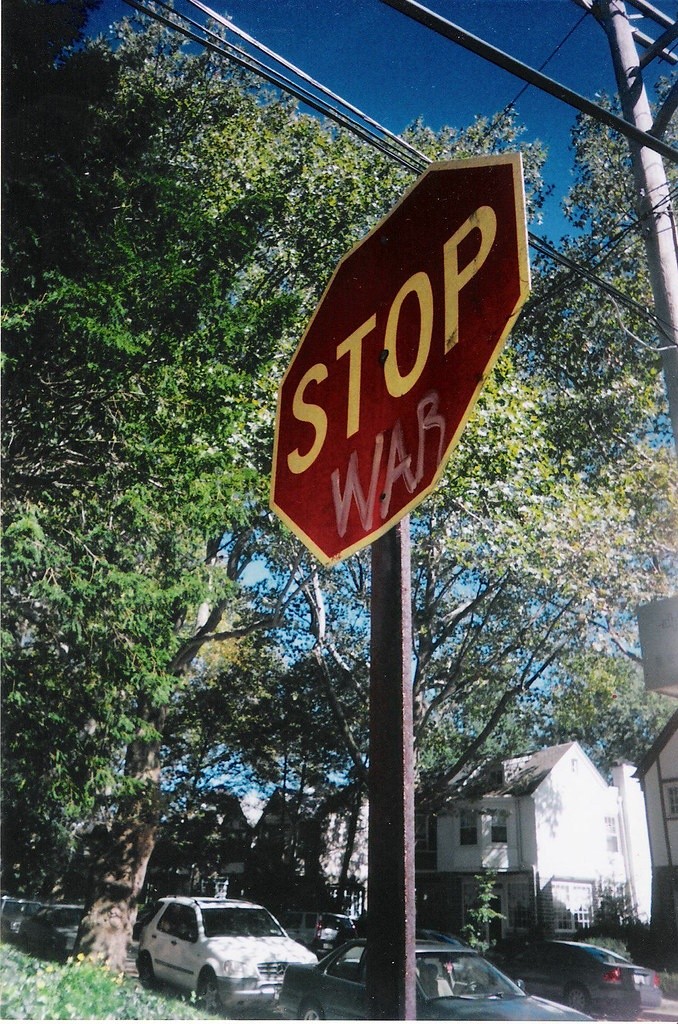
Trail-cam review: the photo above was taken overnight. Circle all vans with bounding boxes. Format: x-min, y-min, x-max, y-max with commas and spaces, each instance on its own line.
270, 909, 359, 961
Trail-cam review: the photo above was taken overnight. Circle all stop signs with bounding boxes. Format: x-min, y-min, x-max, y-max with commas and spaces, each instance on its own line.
268, 149, 533, 571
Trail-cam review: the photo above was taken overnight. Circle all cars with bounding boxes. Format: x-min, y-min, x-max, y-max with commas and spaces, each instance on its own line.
494, 940, 663, 1021
275, 935, 595, 1021
0, 898, 43, 940
16, 904, 87, 963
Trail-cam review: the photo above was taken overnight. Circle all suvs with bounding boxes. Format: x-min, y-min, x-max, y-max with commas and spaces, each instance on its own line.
134, 894, 321, 1016
